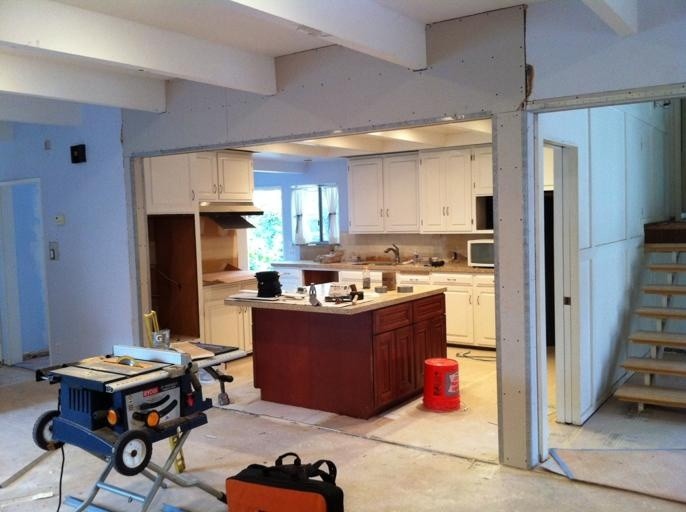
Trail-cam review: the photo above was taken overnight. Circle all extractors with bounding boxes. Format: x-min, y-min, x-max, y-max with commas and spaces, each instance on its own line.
199, 201, 264, 215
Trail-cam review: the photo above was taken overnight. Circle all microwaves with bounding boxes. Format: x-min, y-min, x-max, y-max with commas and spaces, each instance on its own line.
466, 239, 495, 268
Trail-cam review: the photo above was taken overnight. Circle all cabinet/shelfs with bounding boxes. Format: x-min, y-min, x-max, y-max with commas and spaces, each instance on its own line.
339, 270, 383, 283
251, 293, 446, 420
198, 151, 254, 202
142, 151, 199, 215
398, 273, 430, 284
429, 273, 497, 349
473, 145, 492, 197
419, 145, 487, 234
347, 150, 419, 235
203, 278, 258, 355
271, 265, 334, 294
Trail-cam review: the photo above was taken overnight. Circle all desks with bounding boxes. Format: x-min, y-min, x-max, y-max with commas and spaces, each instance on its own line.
291, 244, 339, 261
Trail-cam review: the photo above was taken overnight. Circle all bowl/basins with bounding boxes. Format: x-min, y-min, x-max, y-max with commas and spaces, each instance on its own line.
432, 261, 445, 267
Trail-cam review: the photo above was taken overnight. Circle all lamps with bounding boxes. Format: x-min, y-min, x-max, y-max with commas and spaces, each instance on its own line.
654, 99, 672, 109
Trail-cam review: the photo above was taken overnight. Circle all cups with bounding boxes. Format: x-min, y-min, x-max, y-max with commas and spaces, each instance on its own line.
151, 328, 170, 348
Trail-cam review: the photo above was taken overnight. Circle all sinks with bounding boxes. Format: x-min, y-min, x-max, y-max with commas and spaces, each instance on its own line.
353, 263, 394, 266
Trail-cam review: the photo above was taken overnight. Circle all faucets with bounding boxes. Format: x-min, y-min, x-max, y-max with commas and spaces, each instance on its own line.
383, 243, 399, 262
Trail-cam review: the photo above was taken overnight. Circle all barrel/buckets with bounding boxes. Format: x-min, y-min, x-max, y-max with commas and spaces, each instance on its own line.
422, 359, 460, 412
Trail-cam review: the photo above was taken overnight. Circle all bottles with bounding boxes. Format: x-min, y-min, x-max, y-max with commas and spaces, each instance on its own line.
360, 265, 371, 290
